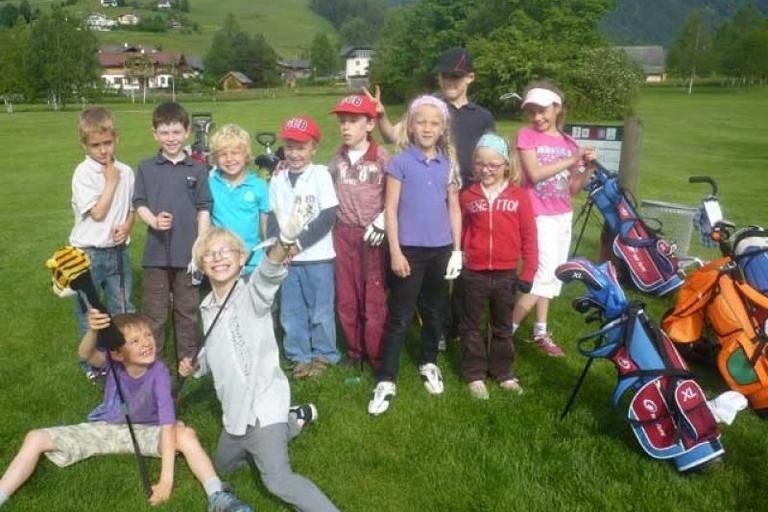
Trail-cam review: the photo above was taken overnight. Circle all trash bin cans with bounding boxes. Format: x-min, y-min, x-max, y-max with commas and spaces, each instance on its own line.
640, 199, 700, 257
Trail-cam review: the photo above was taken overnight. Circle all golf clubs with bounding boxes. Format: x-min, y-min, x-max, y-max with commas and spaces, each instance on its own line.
555, 262, 606, 322
344, 243, 366, 385
170, 239, 278, 401
60, 286, 153, 497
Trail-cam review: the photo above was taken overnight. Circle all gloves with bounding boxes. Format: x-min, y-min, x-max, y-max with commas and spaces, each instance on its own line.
443, 250, 464, 280
519, 279, 533, 291
280, 196, 313, 244
362, 212, 386, 247
185, 259, 204, 285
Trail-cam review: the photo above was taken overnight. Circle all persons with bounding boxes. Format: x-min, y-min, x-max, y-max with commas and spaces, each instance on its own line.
0, 308, 252, 512
364, 96, 463, 416
207, 122, 270, 286
511, 78, 598, 357
271, 117, 341, 379
276, 92, 388, 366
178, 196, 341, 512
68, 106, 136, 380
131, 103, 214, 368
453, 136, 538, 401
361, 47, 501, 352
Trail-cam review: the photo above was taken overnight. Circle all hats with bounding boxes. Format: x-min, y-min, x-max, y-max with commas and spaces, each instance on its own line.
327, 93, 377, 118
520, 88, 562, 109
276, 116, 321, 141
431, 48, 471, 77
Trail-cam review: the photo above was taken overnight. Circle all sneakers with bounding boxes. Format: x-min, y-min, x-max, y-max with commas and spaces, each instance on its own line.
290, 404, 316, 427
286, 352, 356, 381
437, 333, 446, 352
501, 377, 522, 395
208, 481, 251, 512
418, 364, 444, 395
527, 330, 567, 356
468, 380, 488, 398
366, 382, 398, 417
